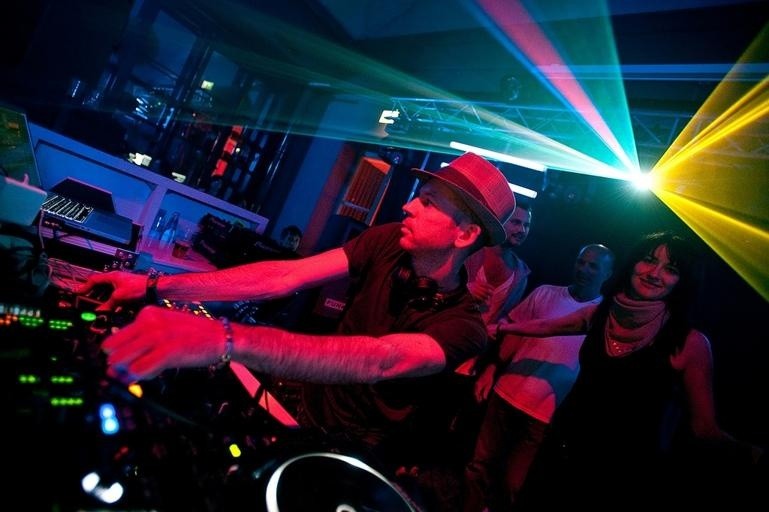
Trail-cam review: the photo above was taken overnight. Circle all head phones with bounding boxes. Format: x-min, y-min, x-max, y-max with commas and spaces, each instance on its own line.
389, 252, 469, 313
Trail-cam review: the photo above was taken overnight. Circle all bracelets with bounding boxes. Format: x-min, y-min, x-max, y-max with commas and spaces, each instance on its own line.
142, 271, 163, 298
494, 320, 503, 334
206, 315, 235, 372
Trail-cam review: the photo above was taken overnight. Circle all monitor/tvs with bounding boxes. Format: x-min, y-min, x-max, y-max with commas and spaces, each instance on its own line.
0, 100, 43, 188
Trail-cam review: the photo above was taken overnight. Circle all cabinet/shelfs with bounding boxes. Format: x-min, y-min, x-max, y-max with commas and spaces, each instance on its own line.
28, 121, 270, 293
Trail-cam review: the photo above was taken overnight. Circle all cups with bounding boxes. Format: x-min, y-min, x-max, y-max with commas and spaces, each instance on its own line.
173, 238, 193, 258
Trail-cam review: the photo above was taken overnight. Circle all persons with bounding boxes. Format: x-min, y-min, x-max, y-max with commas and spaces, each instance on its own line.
444, 240, 616, 509
485, 229, 769, 509
65, 151, 518, 484
279, 225, 303, 253
387, 194, 533, 483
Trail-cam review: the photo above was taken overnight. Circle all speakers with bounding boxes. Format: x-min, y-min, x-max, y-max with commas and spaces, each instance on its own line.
311, 212, 371, 321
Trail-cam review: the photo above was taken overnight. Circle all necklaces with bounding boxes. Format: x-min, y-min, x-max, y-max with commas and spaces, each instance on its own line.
609, 339, 630, 355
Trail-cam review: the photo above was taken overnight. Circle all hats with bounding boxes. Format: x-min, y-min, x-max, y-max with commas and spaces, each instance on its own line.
410, 152, 517, 248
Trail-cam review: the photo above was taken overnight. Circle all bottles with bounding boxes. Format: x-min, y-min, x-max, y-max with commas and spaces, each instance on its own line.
148, 208, 193, 242
